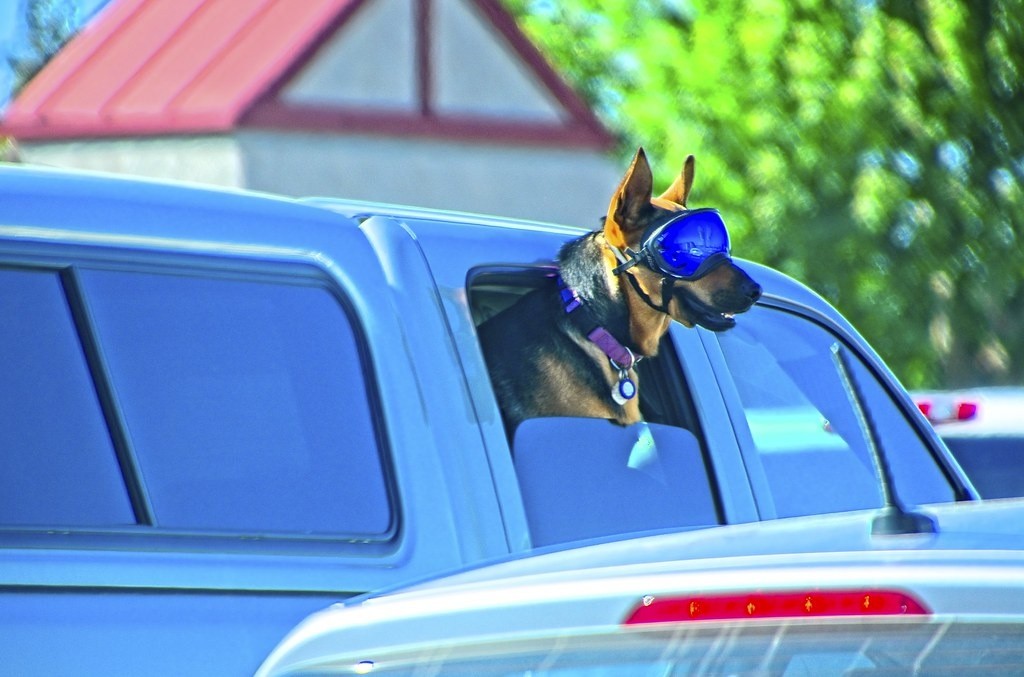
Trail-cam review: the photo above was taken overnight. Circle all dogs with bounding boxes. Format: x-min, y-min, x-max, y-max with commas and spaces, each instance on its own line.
476, 148, 762, 449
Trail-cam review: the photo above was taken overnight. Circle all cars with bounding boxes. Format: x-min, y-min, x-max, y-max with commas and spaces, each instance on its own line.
259, 489, 1022, 677
898, 385, 1024, 500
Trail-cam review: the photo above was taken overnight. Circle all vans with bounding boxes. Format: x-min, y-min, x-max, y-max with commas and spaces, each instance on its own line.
3, 158, 990, 677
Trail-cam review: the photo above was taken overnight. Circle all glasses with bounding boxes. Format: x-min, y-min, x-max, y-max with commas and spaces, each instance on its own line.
640, 206, 731, 278
